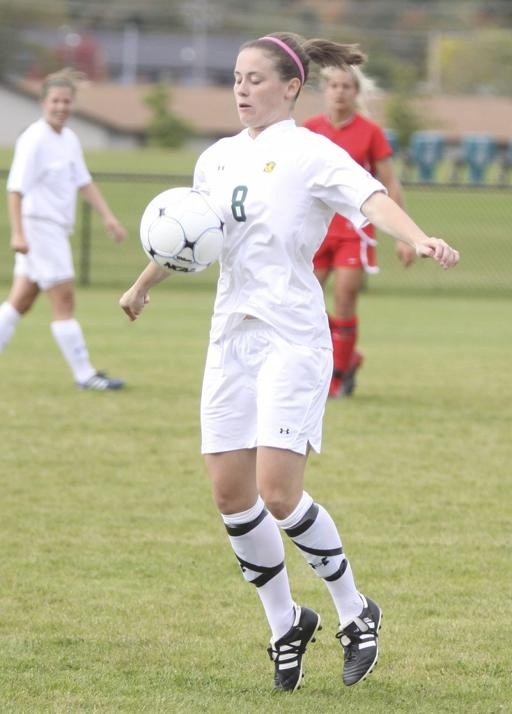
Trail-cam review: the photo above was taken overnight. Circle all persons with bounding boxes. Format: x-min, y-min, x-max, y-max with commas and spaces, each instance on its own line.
120, 32, 462, 695
0, 79, 128, 391
300, 59, 416, 396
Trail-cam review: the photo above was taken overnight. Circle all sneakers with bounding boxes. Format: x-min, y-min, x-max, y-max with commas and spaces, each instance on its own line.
343, 354, 361, 395
268, 604, 323, 694
77, 370, 123, 390
337, 595, 381, 688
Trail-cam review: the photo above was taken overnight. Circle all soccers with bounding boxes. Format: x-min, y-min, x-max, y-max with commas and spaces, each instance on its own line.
140, 188, 223, 274
